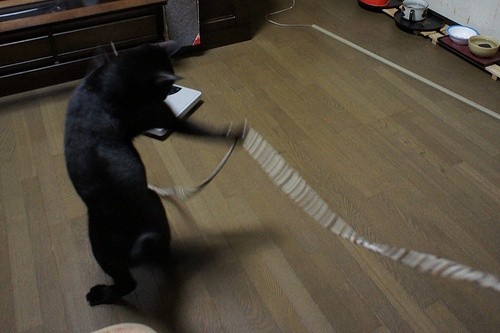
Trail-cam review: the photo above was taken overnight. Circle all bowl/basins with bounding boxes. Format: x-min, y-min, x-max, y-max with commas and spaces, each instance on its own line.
402, 0, 429, 21
446, 25, 500, 58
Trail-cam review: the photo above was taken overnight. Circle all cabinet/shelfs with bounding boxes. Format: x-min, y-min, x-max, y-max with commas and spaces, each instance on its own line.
0, 0, 255, 100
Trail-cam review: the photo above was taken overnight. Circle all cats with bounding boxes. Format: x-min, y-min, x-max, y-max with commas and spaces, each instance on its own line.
63, 39, 282, 332
